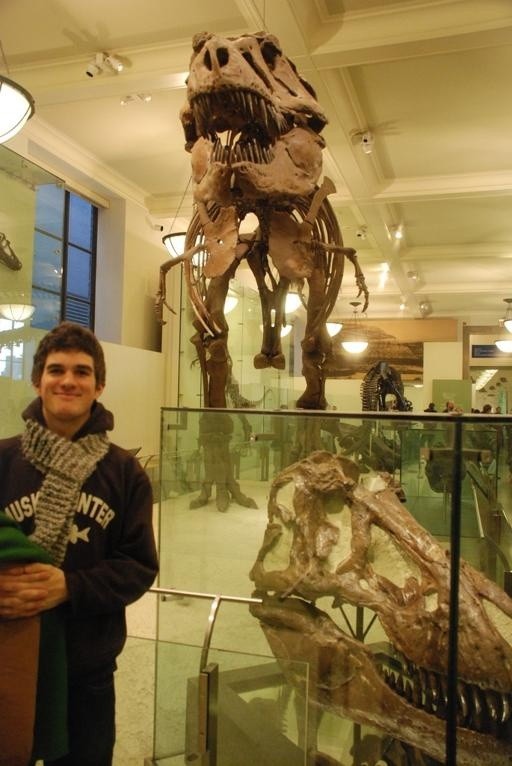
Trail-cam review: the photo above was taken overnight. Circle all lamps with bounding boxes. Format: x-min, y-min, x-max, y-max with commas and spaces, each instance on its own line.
0, 74, 34, 145
325, 302, 369, 355
81, 52, 123, 79
350, 129, 376, 155
495, 300, 511, 356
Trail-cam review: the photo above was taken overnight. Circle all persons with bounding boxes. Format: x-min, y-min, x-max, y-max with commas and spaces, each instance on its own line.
419, 400, 512, 479
0, 319, 161, 764
